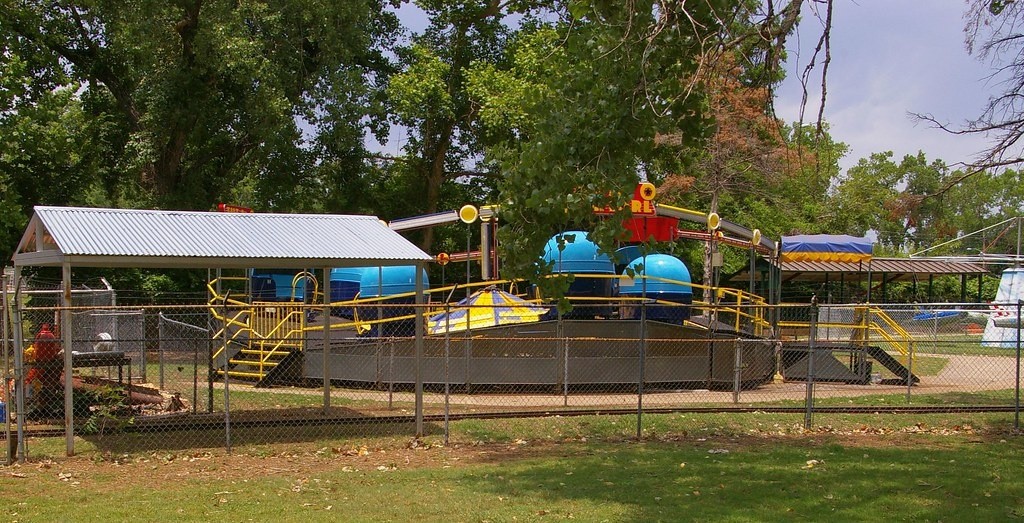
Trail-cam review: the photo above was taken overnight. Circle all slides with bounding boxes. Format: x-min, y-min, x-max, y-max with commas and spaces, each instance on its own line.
866, 344, 919, 380
